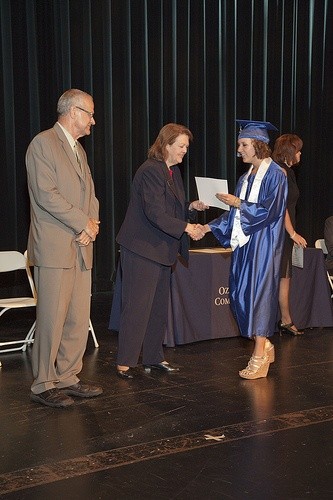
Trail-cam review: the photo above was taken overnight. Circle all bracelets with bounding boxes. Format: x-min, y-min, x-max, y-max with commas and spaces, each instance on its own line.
290, 231, 296, 239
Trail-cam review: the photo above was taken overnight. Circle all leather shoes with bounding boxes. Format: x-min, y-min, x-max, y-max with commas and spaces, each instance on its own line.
31, 387, 74, 407
59, 384, 103, 398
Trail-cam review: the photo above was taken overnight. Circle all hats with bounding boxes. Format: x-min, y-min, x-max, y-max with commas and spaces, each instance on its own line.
234, 120, 278, 145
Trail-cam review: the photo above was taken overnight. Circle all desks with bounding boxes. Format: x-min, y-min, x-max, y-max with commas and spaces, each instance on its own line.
107, 247, 333, 347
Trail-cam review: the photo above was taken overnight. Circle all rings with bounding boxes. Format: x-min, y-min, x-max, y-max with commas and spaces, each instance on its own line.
86, 241, 88, 244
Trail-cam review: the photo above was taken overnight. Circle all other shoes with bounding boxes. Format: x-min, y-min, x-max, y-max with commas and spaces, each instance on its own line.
116, 363, 139, 381
143, 361, 170, 372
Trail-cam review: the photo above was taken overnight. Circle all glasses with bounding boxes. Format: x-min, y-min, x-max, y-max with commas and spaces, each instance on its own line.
75, 106, 93, 117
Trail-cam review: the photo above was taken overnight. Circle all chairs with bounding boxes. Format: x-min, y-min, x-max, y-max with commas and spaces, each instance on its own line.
314, 239, 333, 299
0, 249, 100, 353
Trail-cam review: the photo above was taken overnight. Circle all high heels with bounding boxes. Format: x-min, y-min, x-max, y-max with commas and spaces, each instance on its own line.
279, 320, 304, 337
239, 352, 269, 379
264, 339, 274, 363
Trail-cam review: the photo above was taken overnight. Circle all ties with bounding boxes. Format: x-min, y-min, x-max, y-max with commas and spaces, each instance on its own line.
73, 145, 82, 173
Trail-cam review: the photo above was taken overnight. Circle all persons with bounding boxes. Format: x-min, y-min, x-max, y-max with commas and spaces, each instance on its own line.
262, 133, 308, 338
323, 216, 333, 306
108, 120, 212, 379
188, 120, 288, 381
24, 89, 105, 407
